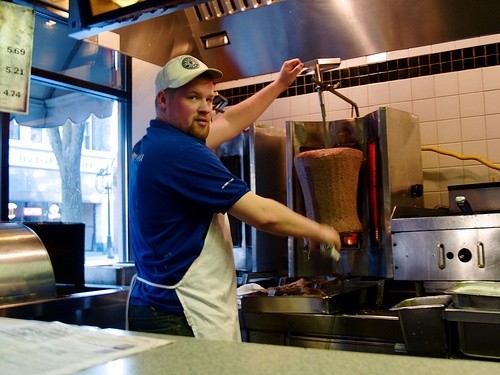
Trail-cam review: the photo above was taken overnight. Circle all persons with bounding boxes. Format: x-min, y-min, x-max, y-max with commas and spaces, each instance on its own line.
125, 53, 341, 342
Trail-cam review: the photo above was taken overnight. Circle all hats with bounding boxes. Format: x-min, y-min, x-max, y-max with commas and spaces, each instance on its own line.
154, 54, 223, 100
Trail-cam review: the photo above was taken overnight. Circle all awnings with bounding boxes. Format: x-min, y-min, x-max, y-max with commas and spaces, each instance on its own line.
8, 8, 118, 129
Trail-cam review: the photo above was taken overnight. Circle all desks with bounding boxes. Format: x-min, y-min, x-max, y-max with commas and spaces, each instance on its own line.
0, 317, 500, 375
